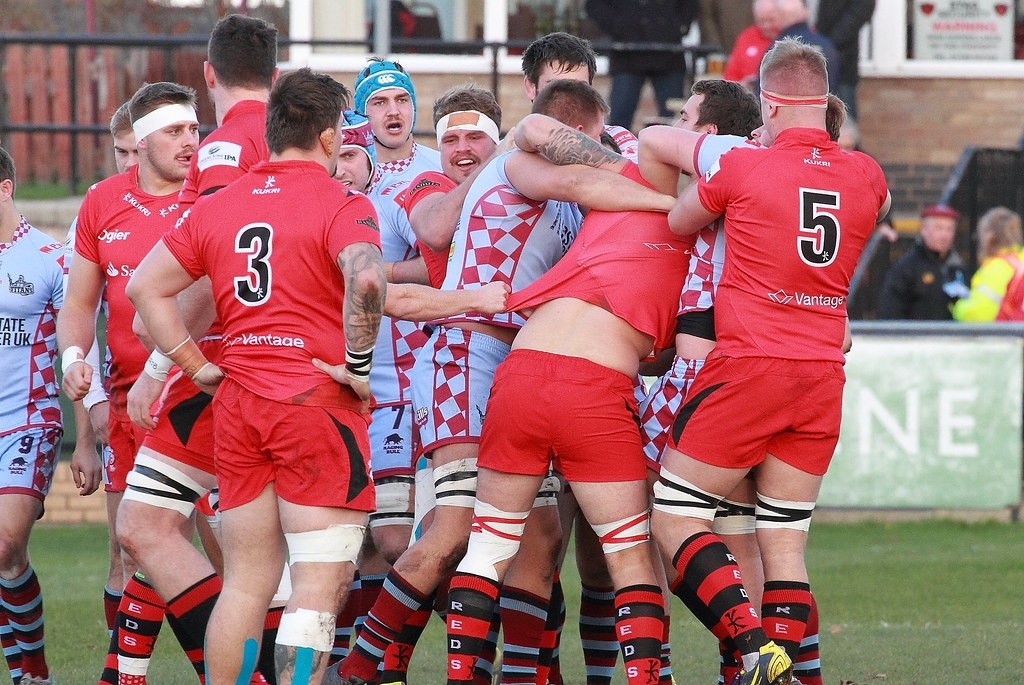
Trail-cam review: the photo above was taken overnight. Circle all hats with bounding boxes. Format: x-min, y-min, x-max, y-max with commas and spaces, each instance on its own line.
920, 206, 958, 220
354, 60, 416, 136
341, 109, 374, 186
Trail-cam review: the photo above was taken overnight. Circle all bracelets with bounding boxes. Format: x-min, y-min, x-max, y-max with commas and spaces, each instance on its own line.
385, 261, 395, 284
345, 345, 375, 382
61, 346, 85, 372
164, 336, 211, 379
144, 350, 176, 382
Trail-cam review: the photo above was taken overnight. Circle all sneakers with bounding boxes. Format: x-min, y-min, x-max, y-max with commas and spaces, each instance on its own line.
323, 656, 368, 685
732, 640, 795, 685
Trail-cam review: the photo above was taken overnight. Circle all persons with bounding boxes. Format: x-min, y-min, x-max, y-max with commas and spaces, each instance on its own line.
879, 205, 968, 319
755, 0, 840, 97
724, 0, 779, 83
56, 81, 200, 639
650, 38, 893, 685
116, 16, 847, 685
62, 103, 139, 444
584, 0, 701, 128
803, 0, 876, 115
953, 208, 1024, 324
0, 148, 102, 685
